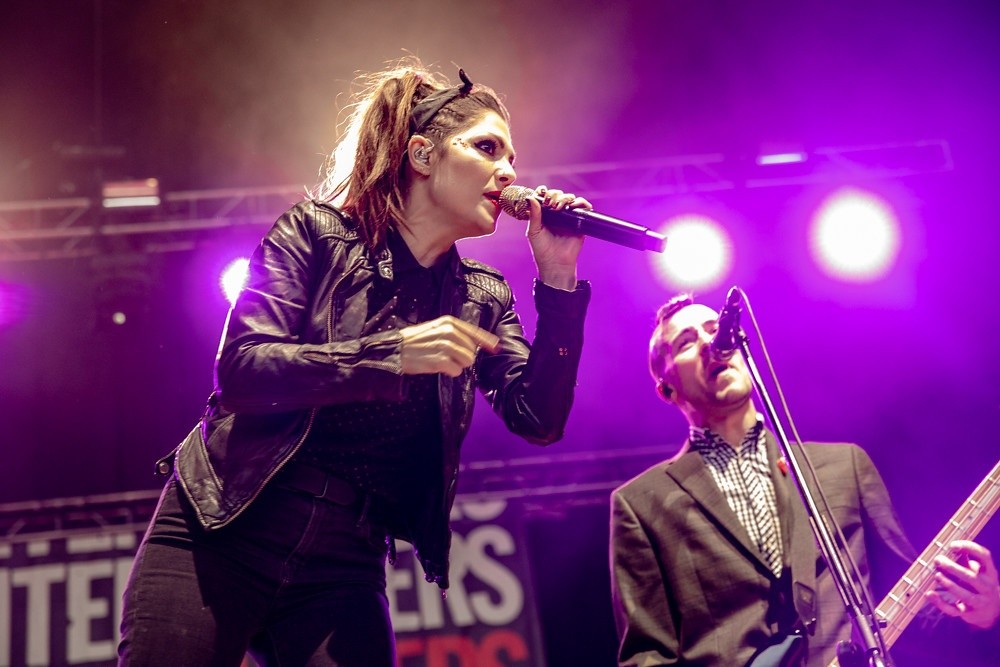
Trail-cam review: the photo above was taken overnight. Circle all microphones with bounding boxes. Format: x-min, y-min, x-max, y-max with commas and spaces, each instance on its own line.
499, 186, 666, 253
712, 287, 744, 361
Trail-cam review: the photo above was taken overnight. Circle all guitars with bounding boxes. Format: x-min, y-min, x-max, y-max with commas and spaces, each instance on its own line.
742, 456, 1000, 667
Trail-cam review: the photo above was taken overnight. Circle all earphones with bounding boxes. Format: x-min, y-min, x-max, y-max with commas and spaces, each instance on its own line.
419, 144, 434, 158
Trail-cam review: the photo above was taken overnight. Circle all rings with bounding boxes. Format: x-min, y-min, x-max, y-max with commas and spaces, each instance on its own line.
966, 595, 975, 606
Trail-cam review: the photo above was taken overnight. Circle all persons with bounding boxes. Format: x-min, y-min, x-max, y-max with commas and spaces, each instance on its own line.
606, 290, 1000, 667
117, 65, 593, 667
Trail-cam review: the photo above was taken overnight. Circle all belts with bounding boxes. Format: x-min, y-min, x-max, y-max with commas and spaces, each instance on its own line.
286, 467, 361, 517
